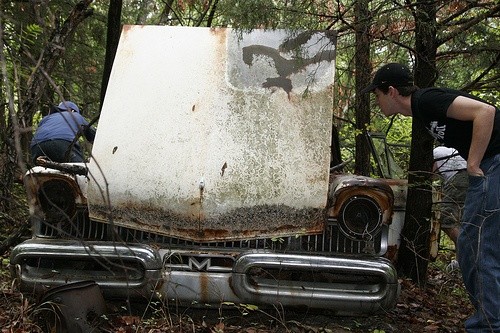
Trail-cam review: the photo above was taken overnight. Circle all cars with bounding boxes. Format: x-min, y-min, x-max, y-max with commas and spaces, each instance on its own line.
9, 23, 443, 316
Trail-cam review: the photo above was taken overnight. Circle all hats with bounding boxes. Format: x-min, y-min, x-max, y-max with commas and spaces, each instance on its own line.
360, 63, 414, 95
56, 101, 79, 113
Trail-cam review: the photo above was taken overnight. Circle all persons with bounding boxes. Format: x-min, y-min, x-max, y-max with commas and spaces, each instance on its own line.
361, 63, 500, 333
433, 146, 468, 244
31, 101, 96, 162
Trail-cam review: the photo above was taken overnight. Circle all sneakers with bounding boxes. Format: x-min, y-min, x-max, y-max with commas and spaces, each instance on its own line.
446, 259, 460, 271
